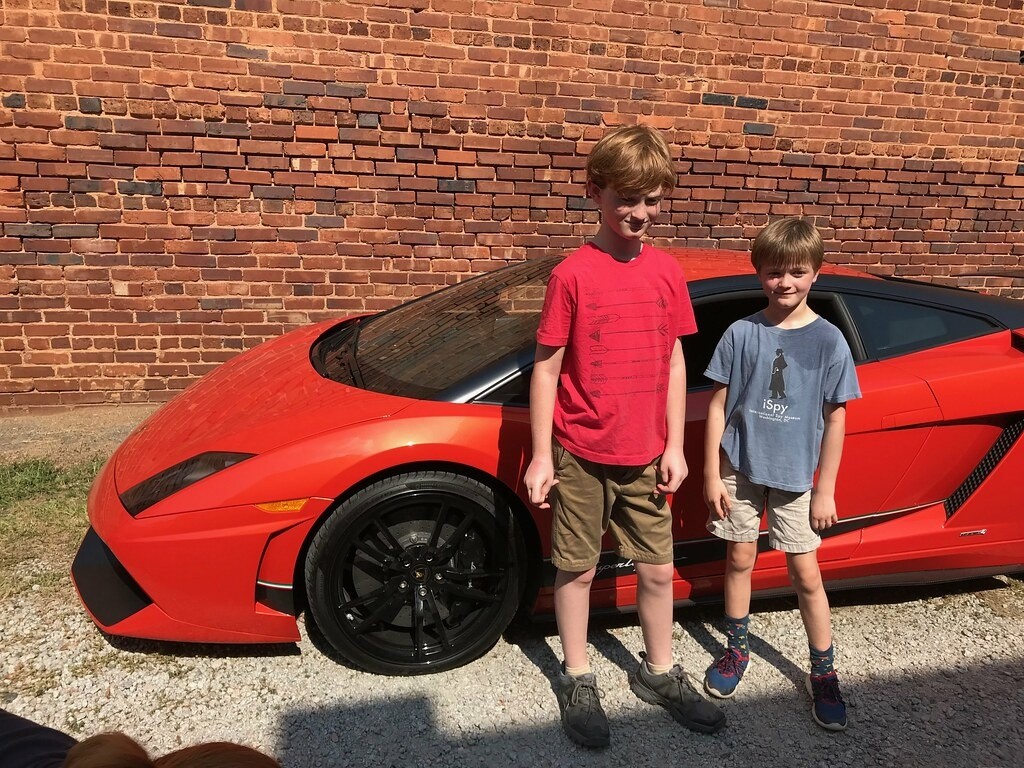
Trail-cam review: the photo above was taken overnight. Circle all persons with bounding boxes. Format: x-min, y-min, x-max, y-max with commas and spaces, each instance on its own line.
522, 122, 728, 751
700, 218, 863, 732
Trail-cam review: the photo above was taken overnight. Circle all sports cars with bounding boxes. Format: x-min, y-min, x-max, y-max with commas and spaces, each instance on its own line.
69, 246, 1023, 678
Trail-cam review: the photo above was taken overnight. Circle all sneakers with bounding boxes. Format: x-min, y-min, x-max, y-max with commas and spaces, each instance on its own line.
556, 660, 610, 746
705, 646, 749, 698
806, 673, 848, 730
630, 651, 726, 732
